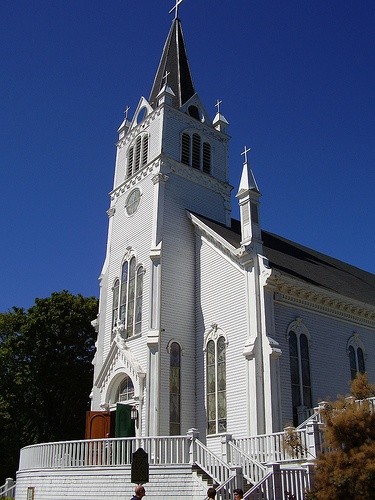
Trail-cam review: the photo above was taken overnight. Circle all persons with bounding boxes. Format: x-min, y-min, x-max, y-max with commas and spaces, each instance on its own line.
207, 488, 217, 500
101, 433, 114, 465
129, 486, 146, 500
233, 489, 246, 500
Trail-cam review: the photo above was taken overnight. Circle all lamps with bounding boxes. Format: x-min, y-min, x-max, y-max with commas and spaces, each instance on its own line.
131, 404, 140, 420
